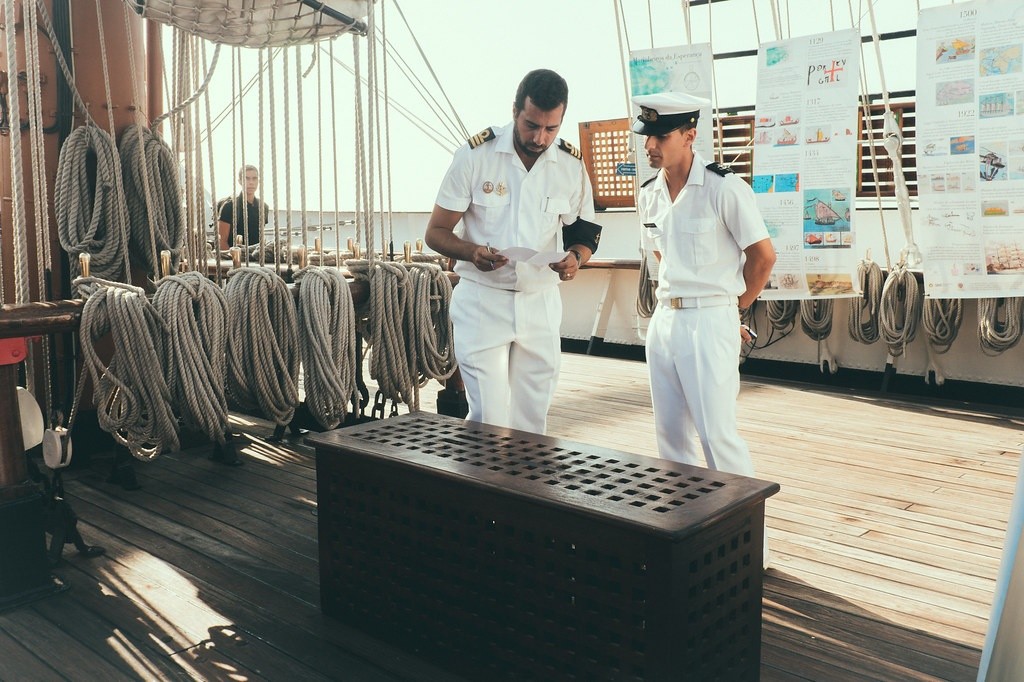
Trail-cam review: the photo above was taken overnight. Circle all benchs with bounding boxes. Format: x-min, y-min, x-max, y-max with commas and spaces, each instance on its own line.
303, 411, 781, 682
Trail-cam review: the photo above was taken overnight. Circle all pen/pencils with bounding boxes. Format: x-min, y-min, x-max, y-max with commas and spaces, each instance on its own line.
485, 242, 496, 271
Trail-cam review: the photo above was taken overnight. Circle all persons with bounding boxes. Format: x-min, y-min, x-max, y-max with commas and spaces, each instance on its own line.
424, 69, 602, 436
217, 165, 269, 251
632, 92, 777, 571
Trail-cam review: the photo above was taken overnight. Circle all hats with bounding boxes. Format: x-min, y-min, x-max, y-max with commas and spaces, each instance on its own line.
630, 91, 711, 136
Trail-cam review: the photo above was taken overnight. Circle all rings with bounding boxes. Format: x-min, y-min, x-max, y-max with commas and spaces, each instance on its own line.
567, 273, 570, 277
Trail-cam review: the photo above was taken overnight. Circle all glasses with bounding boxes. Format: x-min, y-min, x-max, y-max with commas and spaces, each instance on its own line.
244, 177, 259, 182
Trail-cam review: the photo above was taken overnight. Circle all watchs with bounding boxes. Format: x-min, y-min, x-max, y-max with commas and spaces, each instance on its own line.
568, 249, 582, 267
739, 307, 749, 315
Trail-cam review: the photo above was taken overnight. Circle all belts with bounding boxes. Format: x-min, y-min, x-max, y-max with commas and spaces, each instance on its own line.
657, 296, 738, 308
